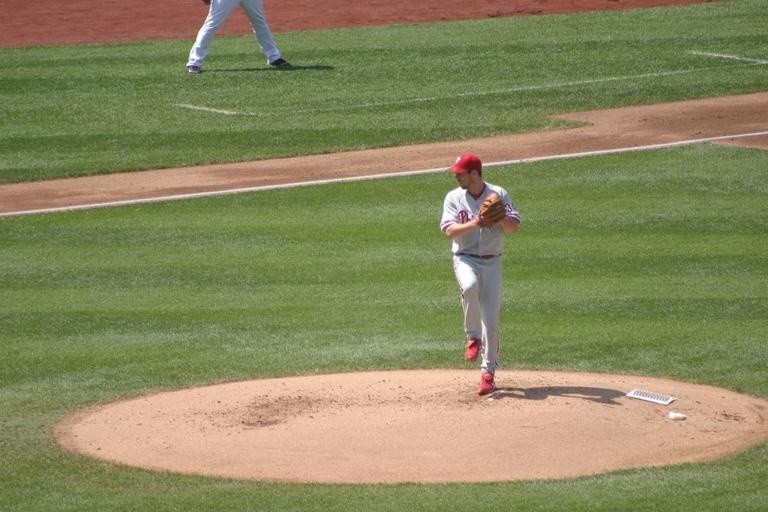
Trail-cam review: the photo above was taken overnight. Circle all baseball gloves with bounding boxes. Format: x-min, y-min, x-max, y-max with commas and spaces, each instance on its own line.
478, 192, 506, 232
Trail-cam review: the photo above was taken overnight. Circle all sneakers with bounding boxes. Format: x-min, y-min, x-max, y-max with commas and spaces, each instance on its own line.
189, 66, 200, 73
478, 370, 496, 396
464, 337, 485, 362
268, 58, 290, 66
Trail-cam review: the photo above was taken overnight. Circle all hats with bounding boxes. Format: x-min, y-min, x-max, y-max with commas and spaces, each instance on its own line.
446, 153, 482, 172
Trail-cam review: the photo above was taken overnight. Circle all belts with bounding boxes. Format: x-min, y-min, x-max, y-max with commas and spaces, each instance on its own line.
452, 252, 502, 259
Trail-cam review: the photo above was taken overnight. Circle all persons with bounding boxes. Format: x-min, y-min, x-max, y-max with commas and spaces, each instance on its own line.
440, 153, 522, 397
186, 0, 291, 74
